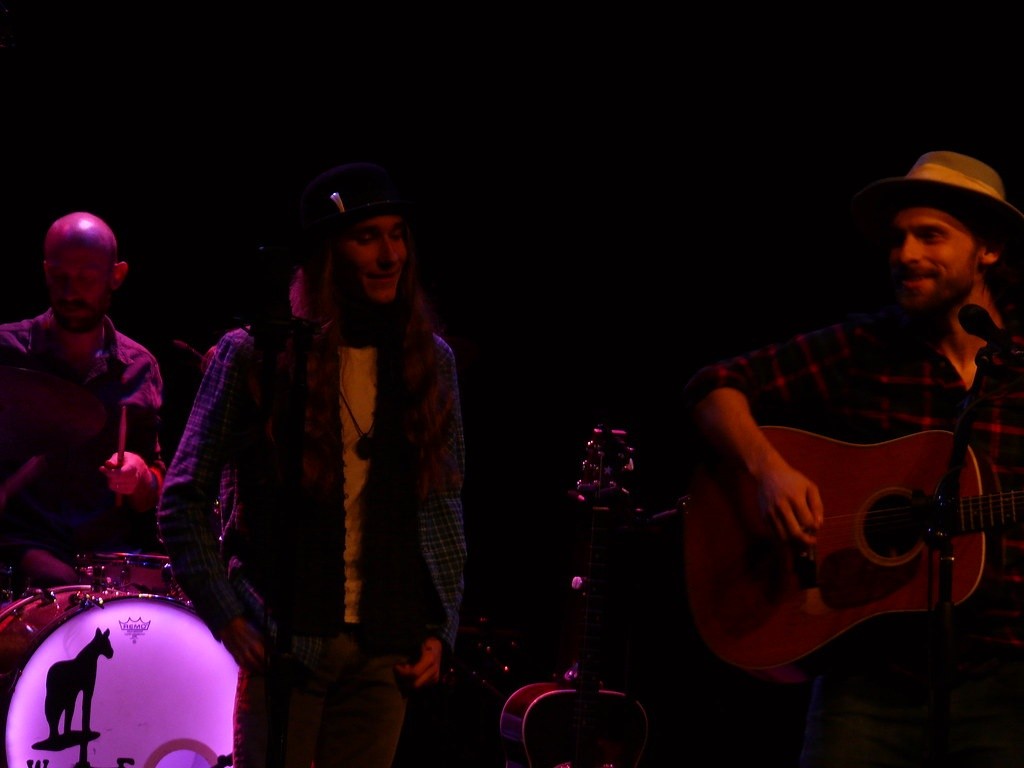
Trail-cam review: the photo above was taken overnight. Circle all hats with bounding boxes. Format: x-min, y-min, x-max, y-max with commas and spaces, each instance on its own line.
849, 150, 1024, 254
301, 162, 405, 246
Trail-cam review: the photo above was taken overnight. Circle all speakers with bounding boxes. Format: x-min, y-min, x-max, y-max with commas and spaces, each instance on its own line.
390, 626, 524, 768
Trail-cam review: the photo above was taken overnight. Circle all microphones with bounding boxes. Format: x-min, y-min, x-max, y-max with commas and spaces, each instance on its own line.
958, 303, 1024, 368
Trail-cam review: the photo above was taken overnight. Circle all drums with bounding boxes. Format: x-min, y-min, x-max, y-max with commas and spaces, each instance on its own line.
0, 582, 241, 768
74, 551, 176, 595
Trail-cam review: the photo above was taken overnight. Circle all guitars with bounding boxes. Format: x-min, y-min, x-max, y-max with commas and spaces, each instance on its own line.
676, 426, 1024, 677
495, 422, 650, 768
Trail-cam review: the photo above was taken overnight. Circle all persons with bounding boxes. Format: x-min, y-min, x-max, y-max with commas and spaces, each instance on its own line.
158, 156, 469, 768
0, 211, 169, 559
681, 153, 1024, 768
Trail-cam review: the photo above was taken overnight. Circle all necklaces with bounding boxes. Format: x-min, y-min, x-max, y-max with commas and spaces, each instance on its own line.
338, 386, 374, 459
